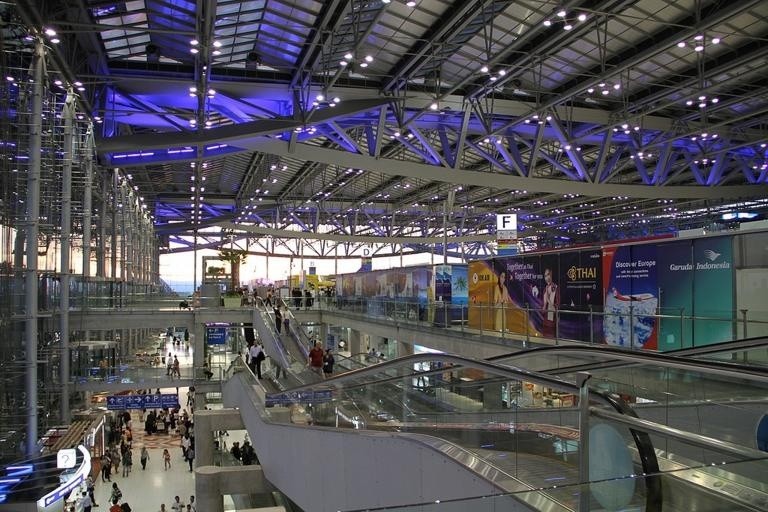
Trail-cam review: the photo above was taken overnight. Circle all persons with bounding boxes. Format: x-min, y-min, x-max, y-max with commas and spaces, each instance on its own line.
283, 307, 295, 335
245, 345, 249, 363
492, 271, 510, 332
189, 495, 196, 512
274, 305, 282, 333
183, 329, 190, 350
109, 500, 121, 512
308, 330, 317, 347
78, 492, 91, 512
415, 363, 425, 386
388, 272, 412, 298
172, 334, 182, 345
234, 287, 258, 305
171, 495, 185, 512
86, 475, 99, 507
366, 346, 384, 363
290, 282, 337, 310
99, 356, 109, 382
95, 387, 258, 483
321, 348, 334, 374
248, 338, 264, 379
304, 402, 313, 426
539, 267, 561, 338
169, 354, 180, 376
159, 503, 166, 512
274, 364, 288, 380
202, 363, 213, 381
119, 503, 131, 512
264, 287, 272, 307
165, 352, 176, 375
559, 438, 569, 453
186, 504, 193, 512
307, 341, 324, 371
337, 339, 346, 348
107, 482, 122, 504
64, 500, 76, 512
